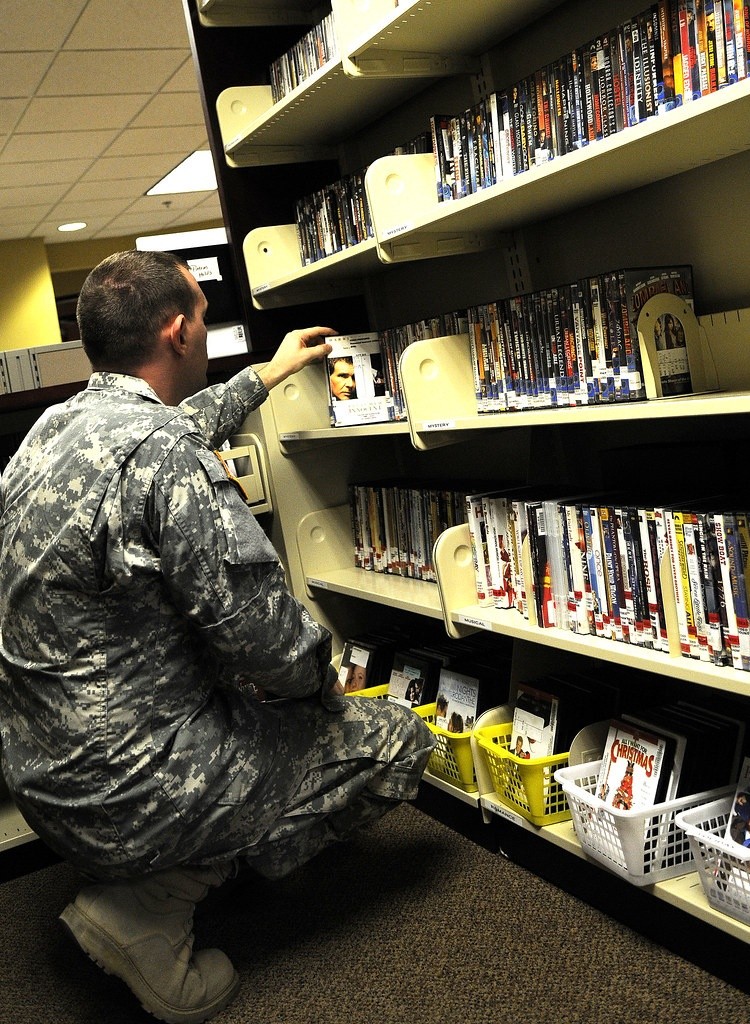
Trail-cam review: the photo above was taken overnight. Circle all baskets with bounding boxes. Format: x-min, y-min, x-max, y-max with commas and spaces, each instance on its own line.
676, 796, 750, 928
476, 723, 573, 827
345, 682, 479, 794
555, 760, 738, 887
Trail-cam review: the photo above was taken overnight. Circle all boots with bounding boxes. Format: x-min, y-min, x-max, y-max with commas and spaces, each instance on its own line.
59, 868, 240, 1024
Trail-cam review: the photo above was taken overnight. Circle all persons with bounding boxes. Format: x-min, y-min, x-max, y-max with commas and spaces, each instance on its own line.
436, 695, 448, 718
350, 665, 366, 693
512, 88, 517, 103
415, 678, 424, 702
665, 314, 679, 350
405, 680, 419, 705
538, 129, 545, 150
590, 53, 597, 71
443, 712, 465, 778
0, 250, 438, 1023
706, 12, 715, 41
507, 736, 530, 795
597, 784, 607, 821
327, 356, 355, 401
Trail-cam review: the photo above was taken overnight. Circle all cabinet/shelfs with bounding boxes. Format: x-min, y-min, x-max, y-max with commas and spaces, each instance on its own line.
181, 0, 750, 997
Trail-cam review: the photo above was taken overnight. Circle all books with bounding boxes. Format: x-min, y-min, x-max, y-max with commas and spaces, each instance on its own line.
325, 264, 697, 429
345, 482, 750, 671
295, 132, 434, 267
337, 623, 750, 913
429, 0, 750, 205
269, 11, 335, 104
